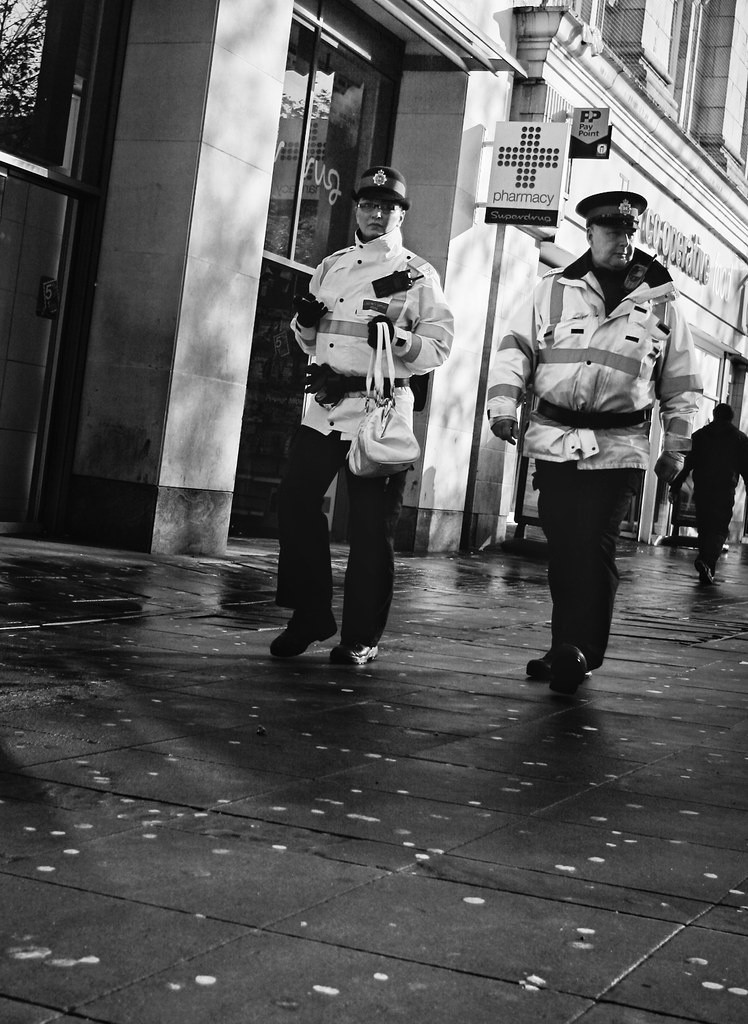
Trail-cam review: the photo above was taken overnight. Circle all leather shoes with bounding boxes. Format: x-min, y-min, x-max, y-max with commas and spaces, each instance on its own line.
549, 644, 588, 694
269, 610, 338, 657
329, 642, 380, 665
526, 648, 592, 678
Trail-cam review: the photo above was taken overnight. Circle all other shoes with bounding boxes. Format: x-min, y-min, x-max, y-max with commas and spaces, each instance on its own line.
694, 559, 714, 583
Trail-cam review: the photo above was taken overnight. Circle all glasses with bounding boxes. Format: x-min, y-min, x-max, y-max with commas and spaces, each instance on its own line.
357, 203, 401, 213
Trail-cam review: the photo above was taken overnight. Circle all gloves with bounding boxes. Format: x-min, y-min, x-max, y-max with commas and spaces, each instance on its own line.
293, 292, 328, 327
366, 315, 394, 349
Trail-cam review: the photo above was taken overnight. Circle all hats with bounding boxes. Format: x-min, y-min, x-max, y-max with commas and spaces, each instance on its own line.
576, 191, 649, 228
351, 166, 412, 210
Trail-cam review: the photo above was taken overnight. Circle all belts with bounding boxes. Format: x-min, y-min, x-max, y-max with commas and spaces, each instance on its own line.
343, 377, 409, 392
538, 398, 650, 429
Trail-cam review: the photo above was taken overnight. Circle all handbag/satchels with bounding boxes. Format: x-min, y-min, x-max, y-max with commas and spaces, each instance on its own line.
345, 322, 422, 478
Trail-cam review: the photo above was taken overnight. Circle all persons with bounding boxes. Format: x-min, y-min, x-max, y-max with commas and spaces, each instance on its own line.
485, 190, 705, 694
668, 404, 747, 584
271, 165, 455, 665
